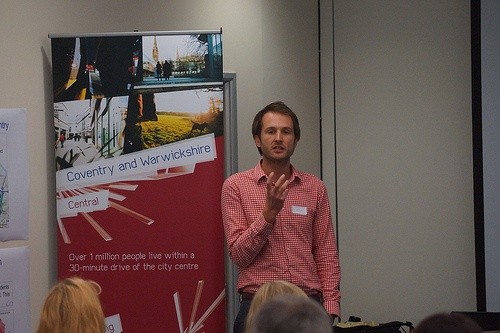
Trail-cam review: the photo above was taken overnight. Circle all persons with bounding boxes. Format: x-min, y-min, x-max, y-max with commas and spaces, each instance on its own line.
59, 58, 175, 149
221, 101, 342, 332
37, 276, 107, 333
412, 312, 483, 333
